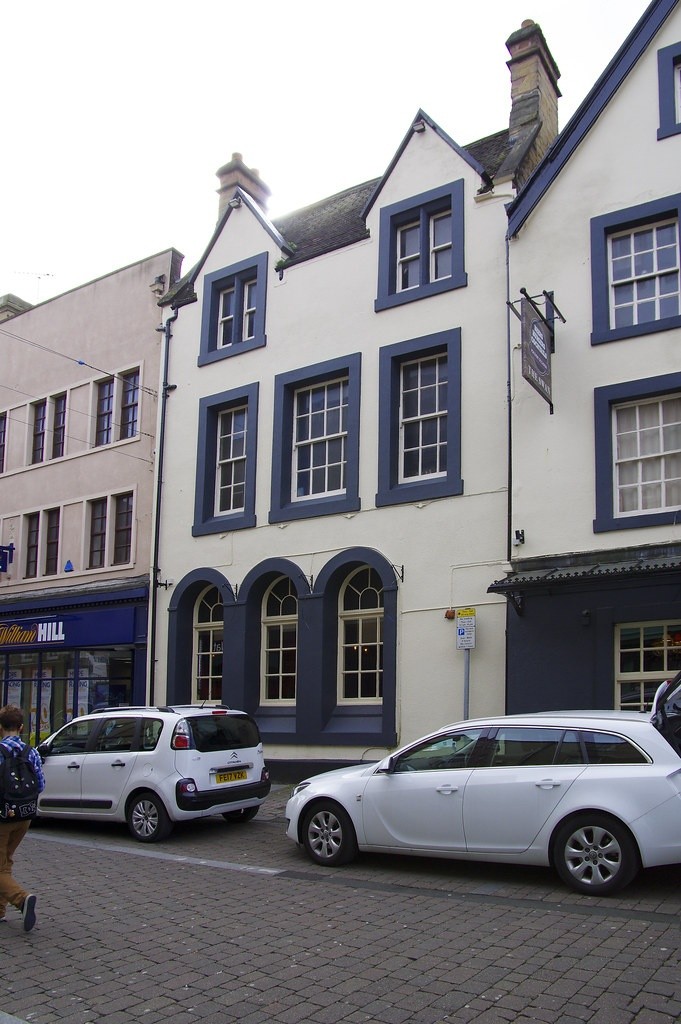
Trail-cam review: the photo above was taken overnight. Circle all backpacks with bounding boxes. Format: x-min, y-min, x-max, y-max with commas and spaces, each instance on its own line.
0, 746, 40, 821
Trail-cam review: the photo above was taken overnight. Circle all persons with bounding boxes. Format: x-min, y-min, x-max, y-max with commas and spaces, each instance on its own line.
0, 705, 45, 931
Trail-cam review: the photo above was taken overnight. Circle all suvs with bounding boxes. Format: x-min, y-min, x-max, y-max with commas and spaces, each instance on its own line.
31, 691, 271, 843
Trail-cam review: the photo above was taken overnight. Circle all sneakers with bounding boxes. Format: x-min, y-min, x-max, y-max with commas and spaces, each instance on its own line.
23, 894, 36, 932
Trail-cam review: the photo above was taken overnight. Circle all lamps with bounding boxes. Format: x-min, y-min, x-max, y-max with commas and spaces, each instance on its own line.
228, 198, 241, 208
412, 121, 425, 133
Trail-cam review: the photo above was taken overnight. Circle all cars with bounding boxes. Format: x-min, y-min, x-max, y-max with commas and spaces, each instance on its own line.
285, 669, 681, 897
